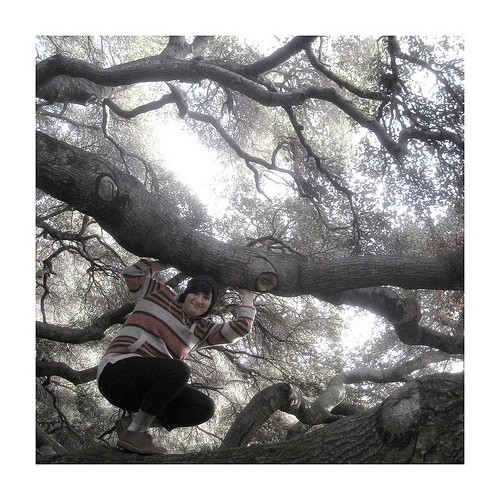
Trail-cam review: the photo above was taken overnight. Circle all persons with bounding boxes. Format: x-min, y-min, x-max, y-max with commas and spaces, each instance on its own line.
95, 258, 256, 454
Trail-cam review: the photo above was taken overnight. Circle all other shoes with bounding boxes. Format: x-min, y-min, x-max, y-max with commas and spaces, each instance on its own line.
116, 413, 135, 440
117, 429, 162, 454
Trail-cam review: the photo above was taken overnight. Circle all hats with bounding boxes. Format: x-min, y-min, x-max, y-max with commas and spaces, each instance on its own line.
187, 275, 219, 318
